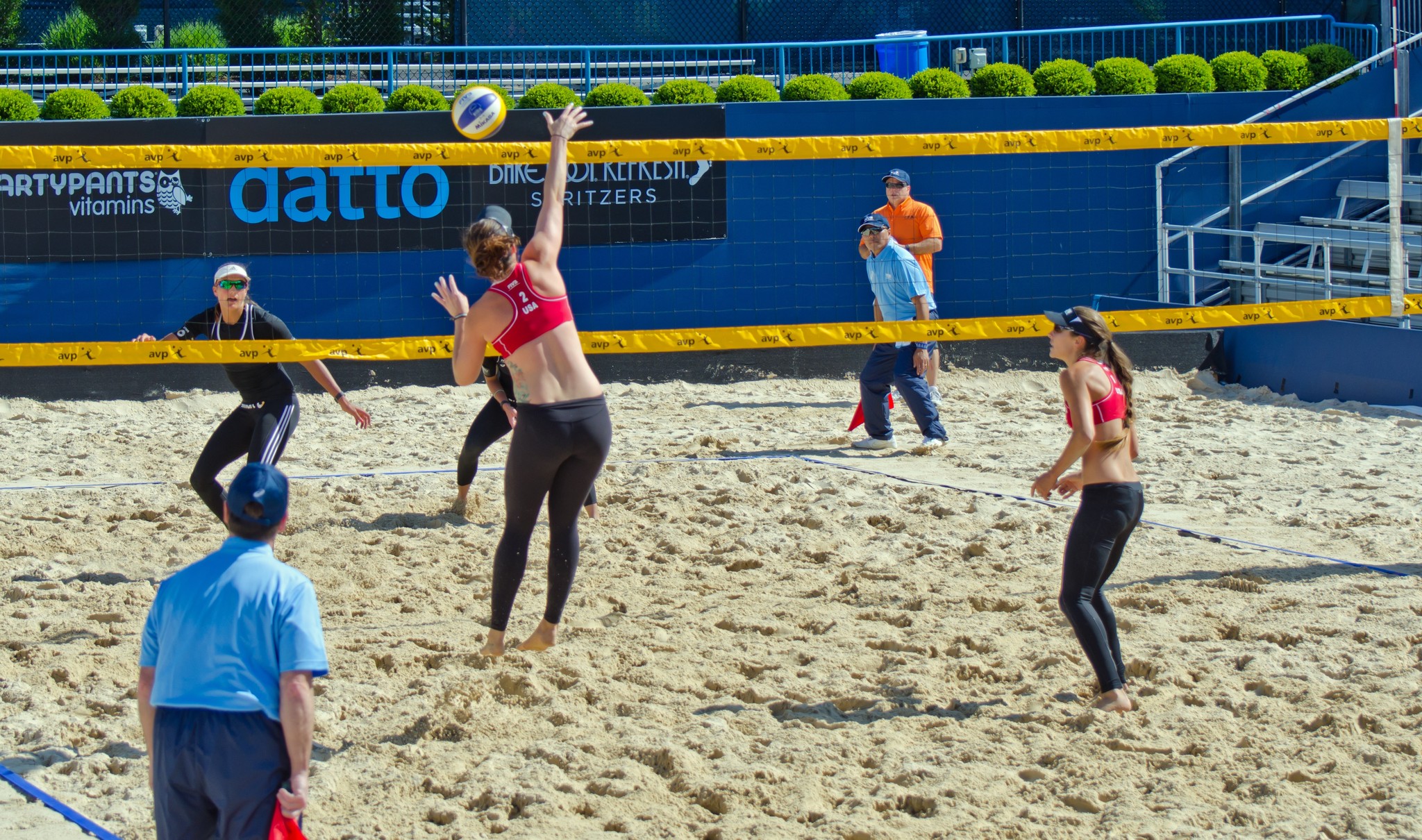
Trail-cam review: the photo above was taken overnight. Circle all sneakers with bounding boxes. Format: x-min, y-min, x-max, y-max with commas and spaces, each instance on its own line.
851, 435, 898, 449
915, 437, 945, 452
929, 389, 943, 407
891, 389, 904, 400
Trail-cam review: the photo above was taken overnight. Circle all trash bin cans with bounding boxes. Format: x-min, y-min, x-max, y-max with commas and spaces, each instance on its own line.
875, 30, 928, 80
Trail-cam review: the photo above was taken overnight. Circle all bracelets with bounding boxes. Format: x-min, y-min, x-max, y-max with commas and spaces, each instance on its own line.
916, 342, 928, 350
551, 133, 568, 140
335, 391, 346, 403
453, 313, 467, 322
500, 399, 510, 410
494, 390, 505, 399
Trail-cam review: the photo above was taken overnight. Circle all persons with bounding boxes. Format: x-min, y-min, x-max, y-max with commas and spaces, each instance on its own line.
859, 168, 943, 407
1031, 306, 1145, 715
457, 341, 601, 521
851, 213, 948, 450
136, 462, 329, 840
131, 262, 371, 532
431, 101, 614, 659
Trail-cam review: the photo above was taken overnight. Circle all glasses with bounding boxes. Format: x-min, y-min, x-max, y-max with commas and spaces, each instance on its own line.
885, 182, 908, 189
215, 280, 247, 290
860, 226, 888, 236
1053, 323, 1079, 336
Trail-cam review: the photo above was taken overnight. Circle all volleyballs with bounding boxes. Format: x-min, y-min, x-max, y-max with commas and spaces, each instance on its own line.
451, 85, 507, 140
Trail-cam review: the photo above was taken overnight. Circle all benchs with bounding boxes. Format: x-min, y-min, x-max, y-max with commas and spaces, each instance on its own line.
1220, 180, 1422, 328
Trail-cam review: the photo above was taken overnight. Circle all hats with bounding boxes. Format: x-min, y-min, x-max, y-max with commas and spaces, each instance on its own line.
475, 205, 512, 236
1043, 307, 1096, 339
226, 462, 289, 526
214, 265, 251, 285
882, 169, 910, 186
858, 213, 890, 233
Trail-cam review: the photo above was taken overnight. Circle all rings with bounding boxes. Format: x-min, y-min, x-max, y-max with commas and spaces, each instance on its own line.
1038, 493, 1043, 496
924, 364, 927, 366
